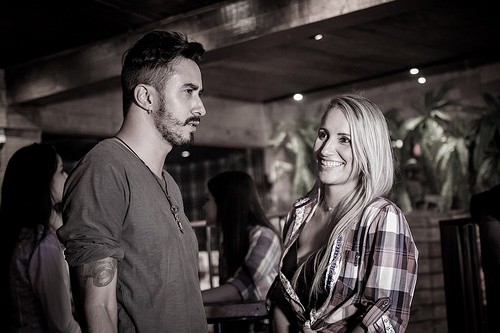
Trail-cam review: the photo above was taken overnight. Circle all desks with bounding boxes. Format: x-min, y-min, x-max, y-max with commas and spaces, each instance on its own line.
203, 302, 267, 333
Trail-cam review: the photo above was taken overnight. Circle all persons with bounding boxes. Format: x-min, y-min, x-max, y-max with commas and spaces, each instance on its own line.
0, 143, 88, 332
56, 30, 206, 333
265, 95, 418, 333
201, 169, 283, 333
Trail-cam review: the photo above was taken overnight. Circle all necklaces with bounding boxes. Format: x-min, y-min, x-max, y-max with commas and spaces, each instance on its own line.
112, 135, 185, 234
323, 199, 342, 212
